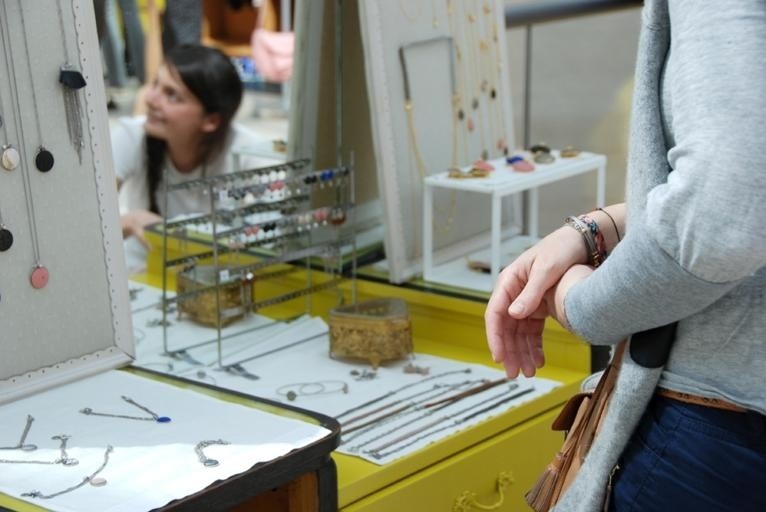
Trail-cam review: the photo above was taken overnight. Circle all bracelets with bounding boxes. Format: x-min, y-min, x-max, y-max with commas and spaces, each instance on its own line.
272, 377, 348, 402
563, 207, 620, 268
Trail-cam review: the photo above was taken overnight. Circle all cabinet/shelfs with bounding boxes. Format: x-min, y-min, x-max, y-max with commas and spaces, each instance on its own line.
125, 221, 594, 512
420, 151, 608, 295
0, 361, 342, 512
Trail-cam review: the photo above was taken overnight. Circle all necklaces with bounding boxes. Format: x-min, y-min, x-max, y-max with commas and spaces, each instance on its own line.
0, 0, 89, 289
0, 432, 80, 465
0, 415, 38, 451
399, 0, 509, 260
78, 394, 171, 424
193, 439, 233, 466
19, 445, 115, 498
331, 369, 535, 460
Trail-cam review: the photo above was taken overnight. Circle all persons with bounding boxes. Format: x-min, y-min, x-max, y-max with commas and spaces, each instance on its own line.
107, 43, 286, 273
483, 0, 765, 511
